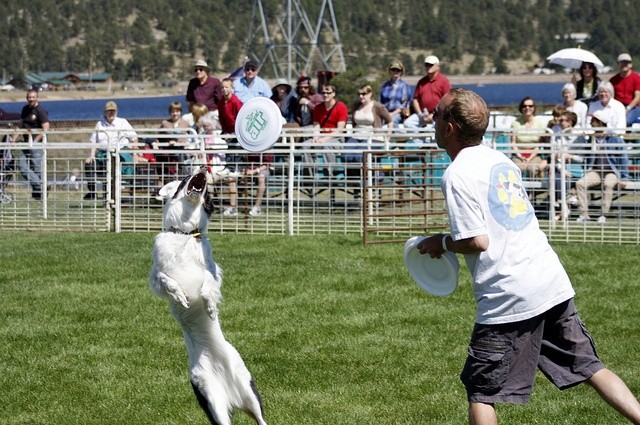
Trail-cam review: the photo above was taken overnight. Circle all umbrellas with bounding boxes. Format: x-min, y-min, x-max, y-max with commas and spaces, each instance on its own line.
545, 44, 605, 84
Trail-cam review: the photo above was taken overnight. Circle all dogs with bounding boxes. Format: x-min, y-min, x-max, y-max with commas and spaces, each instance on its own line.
149, 164, 267, 425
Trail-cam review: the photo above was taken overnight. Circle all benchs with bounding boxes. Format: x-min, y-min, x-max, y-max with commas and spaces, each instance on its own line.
520, 133, 639, 218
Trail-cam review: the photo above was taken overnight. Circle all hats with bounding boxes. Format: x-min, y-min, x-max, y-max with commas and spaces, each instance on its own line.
390, 63, 401, 70
192, 59, 211, 73
271, 78, 292, 95
424, 55, 440, 64
245, 61, 257, 68
588, 110, 608, 123
617, 53, 632, 63
105, 101, 118, 112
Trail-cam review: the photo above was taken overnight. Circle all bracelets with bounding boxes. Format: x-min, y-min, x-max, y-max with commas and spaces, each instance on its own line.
441, 235, 451, 253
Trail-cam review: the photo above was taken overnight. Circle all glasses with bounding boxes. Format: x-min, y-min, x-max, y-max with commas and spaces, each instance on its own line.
560, 119, 567, 122
357, 93, 365, 95
321, 92, 332, 94
582, 65, 593, 70
521, 105, 535, 108
433, 107, 454, 125
246, 68, 255, 71
194, 68, 203, 72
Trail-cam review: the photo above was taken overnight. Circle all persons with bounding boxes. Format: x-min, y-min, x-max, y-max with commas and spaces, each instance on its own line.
141, 138, 176, 193
268, 79, 302, 149
574, 110, 632, 225
547, 105, 567, 151
576, 59, 602, 105
510, 96, 548, 200
588, 81, 626, 146
608, 52, 640, 145
417, 86, 639, 424
558, 82, 588, 134
0, 112, 44, 200
184, 116, 232, 201
341, 77, 394, 188
184, 59, 225, 114
302, 83, 349, 195
222, 148, 273, 217
83, 100, 138, 207
544, 112, 587, 221
181, 100, 210, 144
15, 88, 52, 199
380, 59, 414, 139
159, 102, 192, 168
218, 78, 244, 161
232, 61, 274, 110
296, 75, 324, 138
402, 54, 453, 148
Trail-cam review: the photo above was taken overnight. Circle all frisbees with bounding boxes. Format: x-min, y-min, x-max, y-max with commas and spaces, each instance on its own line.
235, 97, 282, 153
404, 236, 459, 271
404, 235, 459, 296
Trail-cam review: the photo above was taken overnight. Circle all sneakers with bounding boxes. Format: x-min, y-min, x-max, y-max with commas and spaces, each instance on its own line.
576, 215, 591, 221
84, 193, 95, 200
597, 215, 606, 224
223, 206, 237, 217
249, 205, 262, 216
